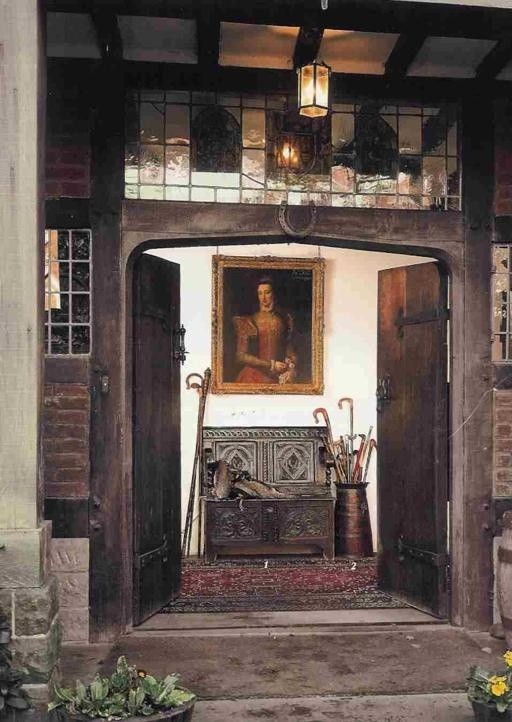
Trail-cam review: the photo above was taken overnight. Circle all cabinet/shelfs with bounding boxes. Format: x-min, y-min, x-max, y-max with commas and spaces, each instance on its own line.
199, 493, 338, 567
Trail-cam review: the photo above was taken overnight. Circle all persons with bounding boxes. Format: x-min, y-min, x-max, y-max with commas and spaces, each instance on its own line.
231, 276, 297, 384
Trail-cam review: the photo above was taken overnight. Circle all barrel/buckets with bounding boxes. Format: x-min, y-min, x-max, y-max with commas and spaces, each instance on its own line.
496, 511, 512, 654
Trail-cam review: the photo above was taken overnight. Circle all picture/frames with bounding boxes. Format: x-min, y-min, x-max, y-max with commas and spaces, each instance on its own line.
206, 250, 326, 398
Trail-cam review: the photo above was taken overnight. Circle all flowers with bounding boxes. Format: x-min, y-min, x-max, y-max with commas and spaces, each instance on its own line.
463, 650, 512, 714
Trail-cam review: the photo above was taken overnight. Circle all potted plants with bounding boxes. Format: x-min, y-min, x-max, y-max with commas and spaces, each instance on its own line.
45, 655, 195, 722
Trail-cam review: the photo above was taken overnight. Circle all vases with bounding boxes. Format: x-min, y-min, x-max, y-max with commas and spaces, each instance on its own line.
467, 692, 510, 722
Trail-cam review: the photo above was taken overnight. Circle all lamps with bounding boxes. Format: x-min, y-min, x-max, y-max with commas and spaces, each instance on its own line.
293, 59, 333, 119
